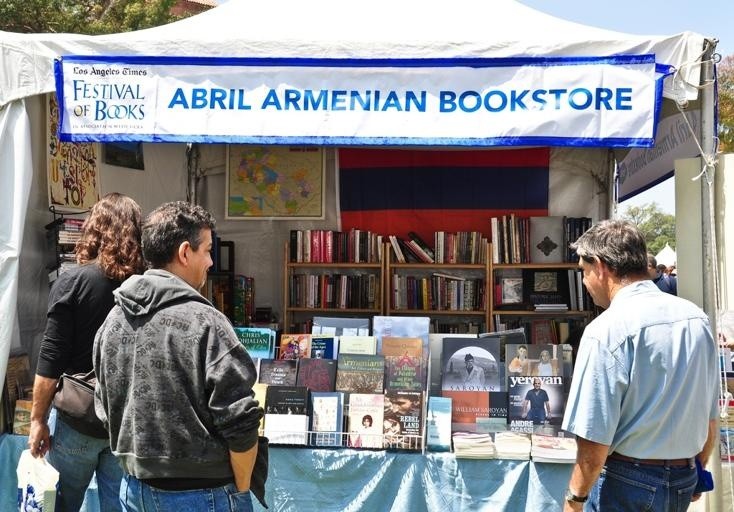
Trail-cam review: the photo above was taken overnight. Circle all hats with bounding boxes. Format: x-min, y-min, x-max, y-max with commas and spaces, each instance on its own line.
464, 354, 473, 361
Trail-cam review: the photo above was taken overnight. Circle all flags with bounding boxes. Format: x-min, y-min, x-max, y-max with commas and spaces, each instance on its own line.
334, 146, 550, 246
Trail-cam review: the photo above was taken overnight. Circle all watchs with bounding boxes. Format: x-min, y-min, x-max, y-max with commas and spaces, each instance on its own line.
563, 486, 589, 504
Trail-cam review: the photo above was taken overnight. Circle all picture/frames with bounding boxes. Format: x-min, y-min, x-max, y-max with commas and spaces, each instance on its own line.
101, 142, 145, 171
224, 143, 326, 221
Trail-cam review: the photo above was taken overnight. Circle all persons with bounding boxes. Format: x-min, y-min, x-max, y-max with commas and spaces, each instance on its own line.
92, 199, 272, 512
560, 216, 721, 512
647, 254, 677, 296
23, 191, 144, 509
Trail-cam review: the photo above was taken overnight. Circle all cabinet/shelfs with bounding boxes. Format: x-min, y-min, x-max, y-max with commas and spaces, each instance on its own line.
49, 205, 95, 277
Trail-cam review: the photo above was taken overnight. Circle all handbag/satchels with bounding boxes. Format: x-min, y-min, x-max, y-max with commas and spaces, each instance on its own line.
51, 372, 96, 420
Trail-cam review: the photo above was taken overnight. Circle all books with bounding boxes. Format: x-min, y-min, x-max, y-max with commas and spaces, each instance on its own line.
198, 212, 605, 463
4, 218, 86, 436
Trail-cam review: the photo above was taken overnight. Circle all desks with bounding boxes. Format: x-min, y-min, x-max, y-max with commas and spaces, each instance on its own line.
1, 433, 574, 512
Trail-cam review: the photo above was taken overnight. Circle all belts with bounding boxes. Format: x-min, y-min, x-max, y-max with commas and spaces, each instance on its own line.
611, 453, 689, 466
